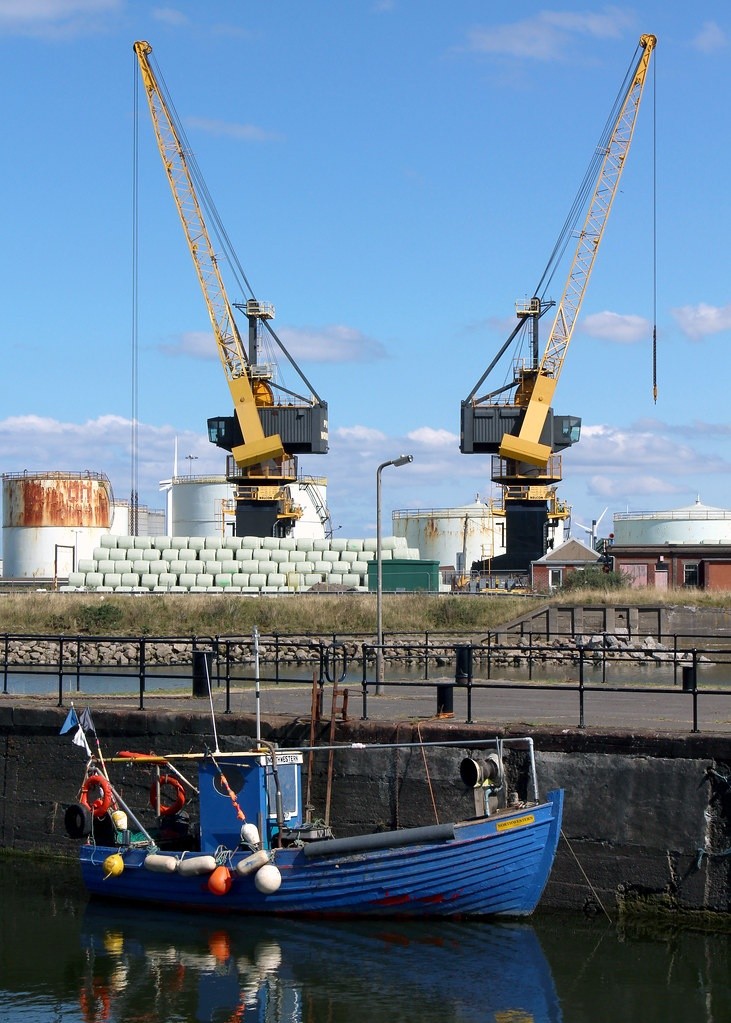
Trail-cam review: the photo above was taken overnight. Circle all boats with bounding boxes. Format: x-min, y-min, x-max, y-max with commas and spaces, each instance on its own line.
63, 629, 566, 925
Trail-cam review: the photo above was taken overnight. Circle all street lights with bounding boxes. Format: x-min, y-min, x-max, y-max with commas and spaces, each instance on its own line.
184, 454, 198, 475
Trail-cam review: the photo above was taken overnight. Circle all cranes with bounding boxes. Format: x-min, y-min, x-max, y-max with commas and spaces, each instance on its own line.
459, 28, 659, 576
134, 39, 332, 539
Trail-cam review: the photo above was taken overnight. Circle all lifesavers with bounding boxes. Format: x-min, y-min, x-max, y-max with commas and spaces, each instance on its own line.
149, 776, 185, 815
64, 803, 91, 838
80, 776, 112, 816
